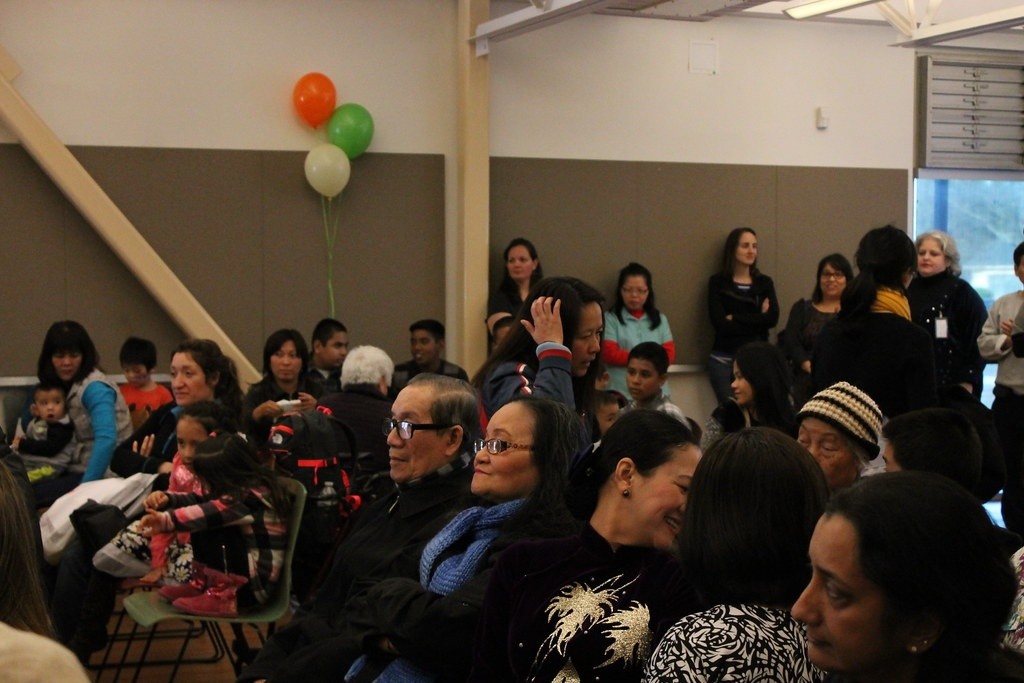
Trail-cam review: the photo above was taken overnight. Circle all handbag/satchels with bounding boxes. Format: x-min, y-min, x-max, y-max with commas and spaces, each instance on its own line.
777, 297, 808, 361
39, 472, 159, 584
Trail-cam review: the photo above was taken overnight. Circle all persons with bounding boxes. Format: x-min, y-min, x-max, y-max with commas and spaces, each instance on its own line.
1, 224, 1024, 683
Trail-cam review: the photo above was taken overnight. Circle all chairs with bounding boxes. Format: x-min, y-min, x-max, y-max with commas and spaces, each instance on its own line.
84, 449, 308, 683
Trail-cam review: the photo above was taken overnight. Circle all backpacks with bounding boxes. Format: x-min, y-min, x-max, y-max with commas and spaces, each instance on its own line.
267, 409, 359, 565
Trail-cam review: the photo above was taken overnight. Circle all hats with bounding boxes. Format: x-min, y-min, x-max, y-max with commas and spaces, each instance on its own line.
796, 381, 884, 460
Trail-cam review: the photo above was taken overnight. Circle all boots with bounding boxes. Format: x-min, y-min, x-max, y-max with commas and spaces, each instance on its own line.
158, 558, 248, 616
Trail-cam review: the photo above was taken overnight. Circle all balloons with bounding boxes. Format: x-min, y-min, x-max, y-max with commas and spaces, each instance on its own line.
293, 72, 337, 130
326, 103, 374, 158
304, 142, 351, 200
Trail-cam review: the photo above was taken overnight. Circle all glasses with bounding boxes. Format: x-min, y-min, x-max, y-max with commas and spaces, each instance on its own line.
474, 438, 530, 455
622, 286, 648, 296
820, 272, 844, 280
381, 417, 450, 440
